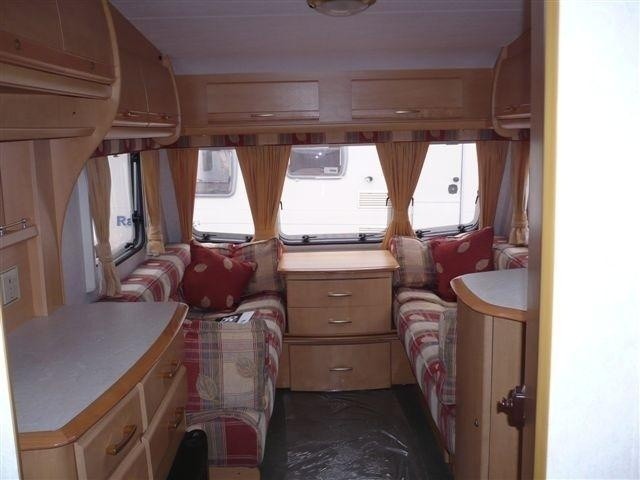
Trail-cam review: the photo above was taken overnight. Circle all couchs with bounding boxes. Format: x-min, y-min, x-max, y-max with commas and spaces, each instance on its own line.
389, 232, 528, 474
93, 234, 287, 479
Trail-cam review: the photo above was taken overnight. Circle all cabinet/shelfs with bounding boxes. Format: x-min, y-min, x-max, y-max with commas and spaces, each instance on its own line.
352, 77, 462, 118
5, 302, 192, 480
493, 47, 530, 122
1, 3, 112, 84
448, 267, 527, 480
111, 7, 177, 128
207, 79, 318, 120
275, 250, 417, 394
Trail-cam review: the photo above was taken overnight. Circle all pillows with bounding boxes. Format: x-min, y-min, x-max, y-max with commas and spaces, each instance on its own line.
178, 237, 257, 312
430, 226, 495, 301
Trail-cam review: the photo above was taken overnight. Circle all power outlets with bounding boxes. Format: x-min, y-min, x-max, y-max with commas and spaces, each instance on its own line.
0, 265, 22, 311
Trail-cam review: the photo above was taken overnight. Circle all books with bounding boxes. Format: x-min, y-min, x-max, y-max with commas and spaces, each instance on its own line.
215, 310, 257, 325
